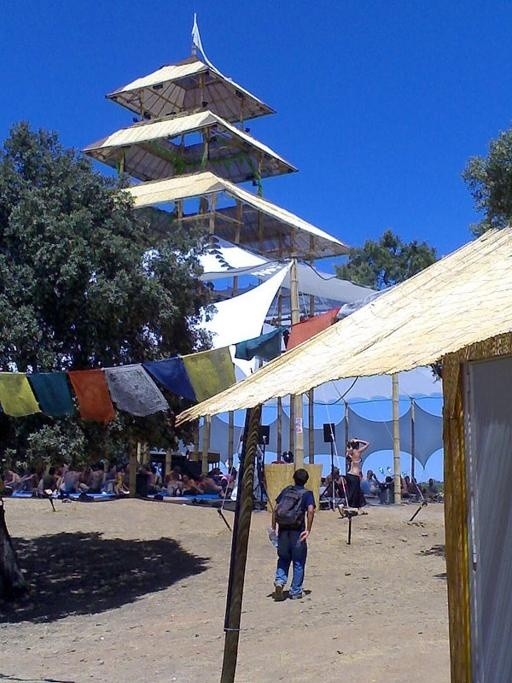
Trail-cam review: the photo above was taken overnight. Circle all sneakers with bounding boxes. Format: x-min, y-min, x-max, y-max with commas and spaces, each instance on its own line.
274, 584, 284, 601
289, 592, 305, 600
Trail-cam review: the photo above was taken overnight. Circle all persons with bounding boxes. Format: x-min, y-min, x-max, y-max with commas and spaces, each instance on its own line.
269, 469, 316, 600
360, 468, 394, 504
324, 467, 340, 489
337, 438, 369, 517
0, 458, 238, 498
404, 475, 443, 501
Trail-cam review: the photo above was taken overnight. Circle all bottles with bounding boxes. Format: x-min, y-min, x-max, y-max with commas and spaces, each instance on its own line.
265, 526, 279, 547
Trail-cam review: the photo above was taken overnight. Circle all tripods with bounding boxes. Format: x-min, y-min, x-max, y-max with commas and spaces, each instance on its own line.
252, 444, 273, 510
319, 442, 347, 511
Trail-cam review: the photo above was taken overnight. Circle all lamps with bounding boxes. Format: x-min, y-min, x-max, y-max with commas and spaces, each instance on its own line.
254, 424, 272, 508
320, 422, 346, 510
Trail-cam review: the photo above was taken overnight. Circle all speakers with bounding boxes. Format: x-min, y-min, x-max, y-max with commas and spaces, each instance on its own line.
324, 424, 335, 442
257, 426, 269, 444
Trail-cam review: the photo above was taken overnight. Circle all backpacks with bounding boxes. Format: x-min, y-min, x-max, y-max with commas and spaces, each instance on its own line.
275, 485, 314, 530
